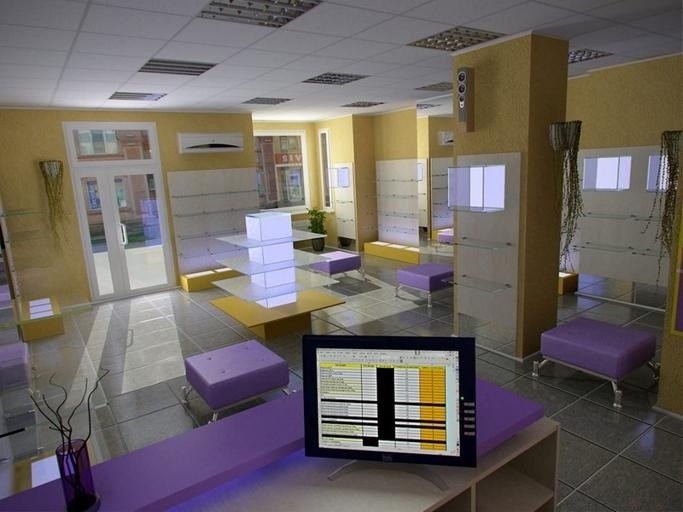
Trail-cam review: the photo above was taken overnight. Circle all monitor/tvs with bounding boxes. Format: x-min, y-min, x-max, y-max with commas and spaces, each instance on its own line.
302, 334, 477, 491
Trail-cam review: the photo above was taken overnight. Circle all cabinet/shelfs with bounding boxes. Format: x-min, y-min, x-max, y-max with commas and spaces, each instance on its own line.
166, 168, 260, 292
0, 207, 94, 341
210, 212, 345, 339
425, 417, 559, 511
447, 167, 511, 293
365, 158, 425, 264
573, 155, 667, 257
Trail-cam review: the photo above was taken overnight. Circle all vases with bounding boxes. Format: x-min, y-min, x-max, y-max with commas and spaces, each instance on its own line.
54, 442, 100, 511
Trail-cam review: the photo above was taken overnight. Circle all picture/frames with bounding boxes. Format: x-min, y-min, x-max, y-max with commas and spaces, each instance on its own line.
670, 223, 683, 336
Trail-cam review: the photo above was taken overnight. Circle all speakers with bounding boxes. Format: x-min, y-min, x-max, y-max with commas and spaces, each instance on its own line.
456, 67, 474, 132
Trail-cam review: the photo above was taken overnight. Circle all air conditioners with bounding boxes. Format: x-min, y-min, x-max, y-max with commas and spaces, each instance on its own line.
177, 132, 244, 154
438, 131, 454, 146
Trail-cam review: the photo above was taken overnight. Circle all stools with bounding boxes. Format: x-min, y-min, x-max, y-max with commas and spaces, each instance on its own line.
396, 263, 453, 308
309, 251, 364, 286
0, 342, 37, 418
439, 230, 454, 244
532, 317, 661, 411
180, 341, 297, 424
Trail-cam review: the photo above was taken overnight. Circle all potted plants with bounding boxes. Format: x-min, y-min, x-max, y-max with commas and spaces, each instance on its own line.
548, 118, 583, 278
304, 208, 327, 251
39, 158, 74, 252
640, 131, 681, 295
